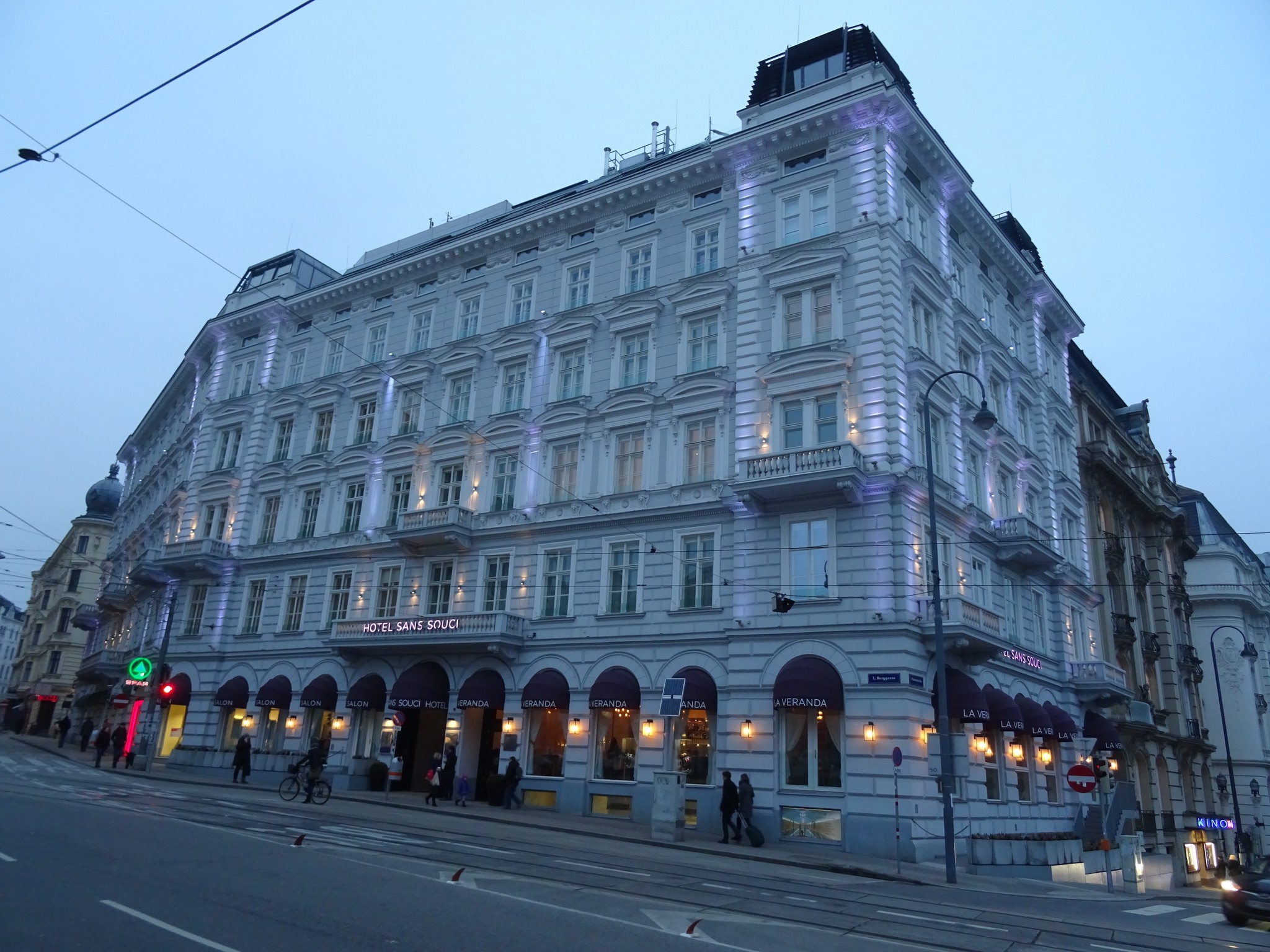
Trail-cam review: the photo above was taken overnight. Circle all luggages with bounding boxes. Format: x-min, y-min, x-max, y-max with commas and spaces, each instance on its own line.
735, 809, 764, 847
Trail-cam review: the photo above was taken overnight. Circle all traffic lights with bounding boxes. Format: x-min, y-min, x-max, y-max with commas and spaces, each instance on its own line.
1099, 756, 1114, 794
1093, 757, 1107, 783
160, 682, 173, 708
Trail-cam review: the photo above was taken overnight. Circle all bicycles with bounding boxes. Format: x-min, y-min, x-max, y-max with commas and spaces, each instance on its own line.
279, 764, 332, 805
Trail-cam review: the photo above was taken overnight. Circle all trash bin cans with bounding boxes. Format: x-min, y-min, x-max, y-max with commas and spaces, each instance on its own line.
388, 757, 404, 780
87, 728, 102, 749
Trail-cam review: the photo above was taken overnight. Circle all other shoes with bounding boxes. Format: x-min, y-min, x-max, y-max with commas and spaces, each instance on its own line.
731, 835, 741, 841
443, 799, 448, 801
447, 798, 452, 801
432, 803, 437, 807
304, 787, 308, 793
233, 780, 238, 783
719, 839, 728, 844
425, 796, 429, 805
241, 780, 249, 784
302, 798, 311, 804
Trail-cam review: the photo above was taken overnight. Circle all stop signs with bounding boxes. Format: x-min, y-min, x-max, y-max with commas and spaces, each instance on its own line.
112, 693, 129, 709
1066, 765, 1097, 793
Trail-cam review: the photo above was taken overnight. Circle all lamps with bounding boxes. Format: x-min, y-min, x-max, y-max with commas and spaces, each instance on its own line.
741, 720, 752, 738
864, 722, 876, 741
1009, 737, 1023, 757
241, 715, 253, 727
1039, 743, 1052, 762
503, 717, 513, 732
642, 719, 654, 736
772, 592, 795, 612
922, 724, 936, 743
974, 729, 988, 751
1106, 757, 1118, 770
332, 716, 343, 729
569, 718, 580, 734
285, 715, 297, 729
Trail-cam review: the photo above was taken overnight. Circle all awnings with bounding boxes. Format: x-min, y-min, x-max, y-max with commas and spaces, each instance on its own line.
345, 673, 387, 711
213, 674, 250, 710
931, 663, 1078, 743
588, 668, 641, 710
521, 669, 570, 710
299, 673, 338, 712
672, 668, 717, 716
154, 671, 192, 706
772, 657, 844, 712
388, 662, 450, 711
457, 669, 506, 711
255, 674, 293, 711
1083, 708, 1123, 750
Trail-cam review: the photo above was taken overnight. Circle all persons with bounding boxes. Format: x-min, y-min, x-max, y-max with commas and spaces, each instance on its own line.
232, 733, 251, 784
717, 771, 755, 844
79, 716, 94, 753
295, 742, 323, 803
454, 772, 471, 807
95, 719, 127, 770
425, 746, 457, 807
1214, 855, 1226, 889
58, 715, 71, 748
1228, 855, 1242, 885
505, 756, 522, 809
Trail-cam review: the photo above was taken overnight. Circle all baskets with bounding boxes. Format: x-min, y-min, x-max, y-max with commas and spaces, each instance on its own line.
288, 765, 300, 774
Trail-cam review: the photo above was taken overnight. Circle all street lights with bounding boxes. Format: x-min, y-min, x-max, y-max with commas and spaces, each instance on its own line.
1210, 625, 1256, 865
923, 370, 998, 886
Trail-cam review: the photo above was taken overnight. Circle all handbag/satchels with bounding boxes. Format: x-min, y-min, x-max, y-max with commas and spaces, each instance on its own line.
427, 769, 433, 779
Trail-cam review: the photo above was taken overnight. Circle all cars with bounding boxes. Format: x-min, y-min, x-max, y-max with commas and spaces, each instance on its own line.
1221, 855, 1270, 926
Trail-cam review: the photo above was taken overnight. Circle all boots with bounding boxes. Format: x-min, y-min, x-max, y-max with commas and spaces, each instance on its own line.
455, 799, 459, 806
462, 801, 467, 806
